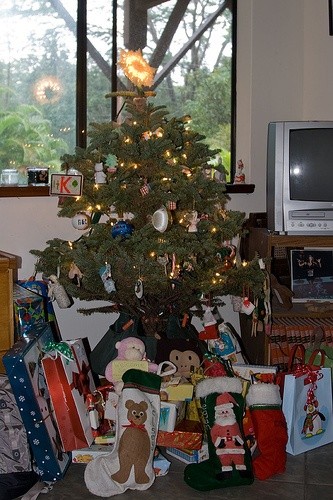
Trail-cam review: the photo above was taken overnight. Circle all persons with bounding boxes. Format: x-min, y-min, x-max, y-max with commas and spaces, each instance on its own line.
297, 255, 322, 286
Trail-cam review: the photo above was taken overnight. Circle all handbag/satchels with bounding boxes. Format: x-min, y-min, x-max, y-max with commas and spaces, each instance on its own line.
276, 343, 325, 402
40, 337, 103, 453
282, 367, 333, 456
302, 326, 333, 380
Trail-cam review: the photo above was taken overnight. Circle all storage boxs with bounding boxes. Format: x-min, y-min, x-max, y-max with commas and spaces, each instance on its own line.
72, 445, 112, 463
154, 377, 209, 464
0, 321, 71, 482
13, 279, 57, 338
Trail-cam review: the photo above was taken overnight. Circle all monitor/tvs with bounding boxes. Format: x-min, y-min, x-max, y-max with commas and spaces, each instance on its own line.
265, 121, 333, 237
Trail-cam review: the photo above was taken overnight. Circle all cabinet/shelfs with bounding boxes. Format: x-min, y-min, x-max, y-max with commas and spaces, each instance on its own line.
244, 227, 333, 366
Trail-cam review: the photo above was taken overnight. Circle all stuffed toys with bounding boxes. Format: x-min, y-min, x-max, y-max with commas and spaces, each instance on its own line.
104, 338, 159, 399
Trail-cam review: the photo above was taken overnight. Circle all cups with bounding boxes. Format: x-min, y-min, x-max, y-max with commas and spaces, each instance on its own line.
1, 169, 19, 186
27, 168, 49, 187
